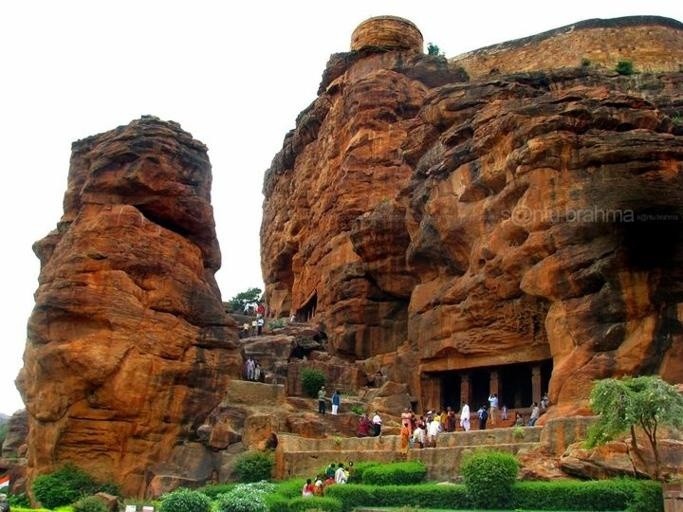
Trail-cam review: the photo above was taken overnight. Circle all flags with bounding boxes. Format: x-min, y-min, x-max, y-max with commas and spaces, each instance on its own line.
0, 473, 12, 493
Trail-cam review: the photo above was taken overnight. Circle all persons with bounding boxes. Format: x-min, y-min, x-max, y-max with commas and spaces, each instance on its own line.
356, 415, 372, 437
372, 410, 383, 437
331, 389, 341, 415
245, 357, 256, 381
290, 313, 297, 322
317, 386, 327, 416
243, 300, 265, 337
400, 392, 550, 454
302, 461, 350, 497
254, 359, 262, 381
